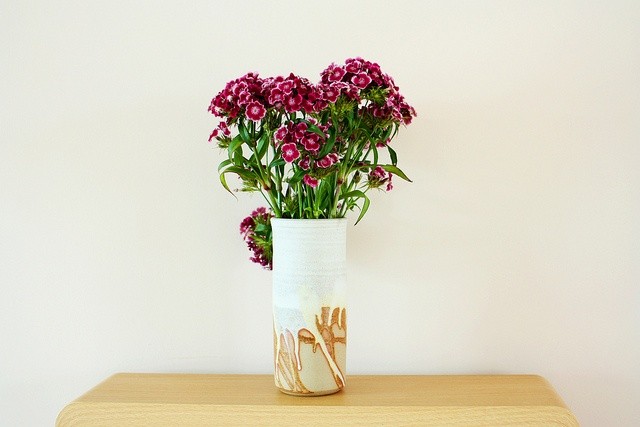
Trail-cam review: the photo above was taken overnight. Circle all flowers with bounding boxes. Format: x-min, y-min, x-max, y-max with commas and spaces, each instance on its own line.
207, 58, 416, 266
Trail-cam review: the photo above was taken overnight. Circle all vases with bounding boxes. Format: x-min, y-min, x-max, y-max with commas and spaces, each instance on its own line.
270, 218, 350, 398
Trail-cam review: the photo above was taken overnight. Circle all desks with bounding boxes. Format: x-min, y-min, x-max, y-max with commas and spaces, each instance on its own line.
57, 374, 579, 425
